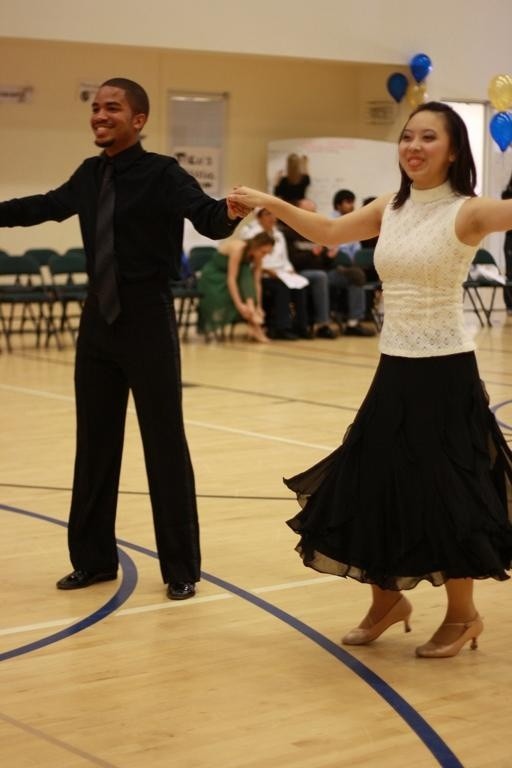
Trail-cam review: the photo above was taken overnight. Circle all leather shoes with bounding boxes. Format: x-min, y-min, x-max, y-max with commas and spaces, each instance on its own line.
345, 324, 374, 336
268, 325, 296, 339
167, 580, 194, 601
317, 324, 334, 338
294, 322, 312, 337
57, 568, 117, 588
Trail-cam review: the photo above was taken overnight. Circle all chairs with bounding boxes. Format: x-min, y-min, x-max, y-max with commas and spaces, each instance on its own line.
1, 244, 512, 356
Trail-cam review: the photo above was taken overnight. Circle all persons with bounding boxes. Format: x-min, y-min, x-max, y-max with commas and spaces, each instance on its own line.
179, 152, 380, 344
0, 79, 255, 600
227, 102, 512, 658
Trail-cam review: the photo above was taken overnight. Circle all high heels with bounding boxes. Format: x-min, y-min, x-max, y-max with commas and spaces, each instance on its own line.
342, 594, 412, 645
416, 611, 483, 658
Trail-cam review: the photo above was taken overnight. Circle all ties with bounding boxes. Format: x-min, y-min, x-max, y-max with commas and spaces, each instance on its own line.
94, 164, 122, 323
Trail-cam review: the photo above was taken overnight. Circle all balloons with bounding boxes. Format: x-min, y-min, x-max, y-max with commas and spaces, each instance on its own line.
405, 78, 427, 111
489, 111, 512, 152
487, 73, 512, 112
409, 53, 431, 83
387, 72, 408, 103
